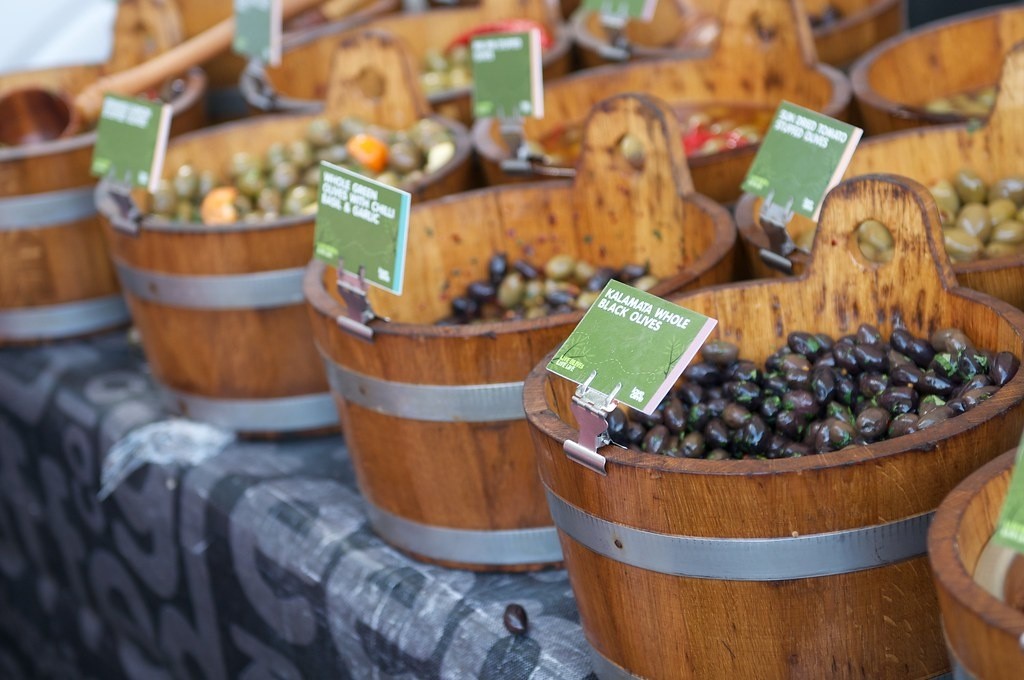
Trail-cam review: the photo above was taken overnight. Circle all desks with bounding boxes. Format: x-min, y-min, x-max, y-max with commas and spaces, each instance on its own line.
0, 326, 599, 680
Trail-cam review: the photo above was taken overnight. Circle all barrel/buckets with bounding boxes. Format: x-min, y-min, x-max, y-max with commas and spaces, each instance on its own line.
0, 1, 1023, 680
